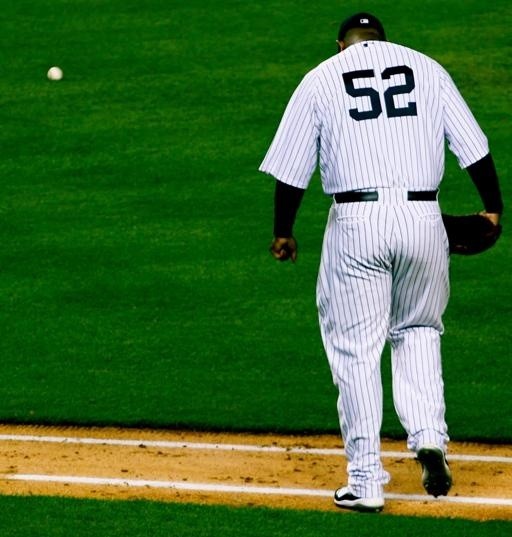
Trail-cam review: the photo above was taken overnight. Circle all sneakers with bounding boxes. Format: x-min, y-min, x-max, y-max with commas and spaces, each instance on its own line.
333, 487, 385, 512
414, 447, 452, 498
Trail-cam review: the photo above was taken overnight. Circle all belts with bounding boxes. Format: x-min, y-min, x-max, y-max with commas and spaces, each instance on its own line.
335, 191, 438, 203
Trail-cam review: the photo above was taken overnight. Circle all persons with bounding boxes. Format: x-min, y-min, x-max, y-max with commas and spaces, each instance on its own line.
258, 11, 507, 515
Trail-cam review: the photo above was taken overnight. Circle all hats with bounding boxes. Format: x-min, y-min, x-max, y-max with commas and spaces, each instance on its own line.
338, 13, 387, 42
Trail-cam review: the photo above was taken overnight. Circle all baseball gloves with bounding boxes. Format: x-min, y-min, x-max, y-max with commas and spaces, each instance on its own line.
443, 213, 503, 254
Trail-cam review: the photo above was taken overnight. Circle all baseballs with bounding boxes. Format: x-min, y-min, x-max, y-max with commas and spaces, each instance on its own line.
47, 67, 62, 80
